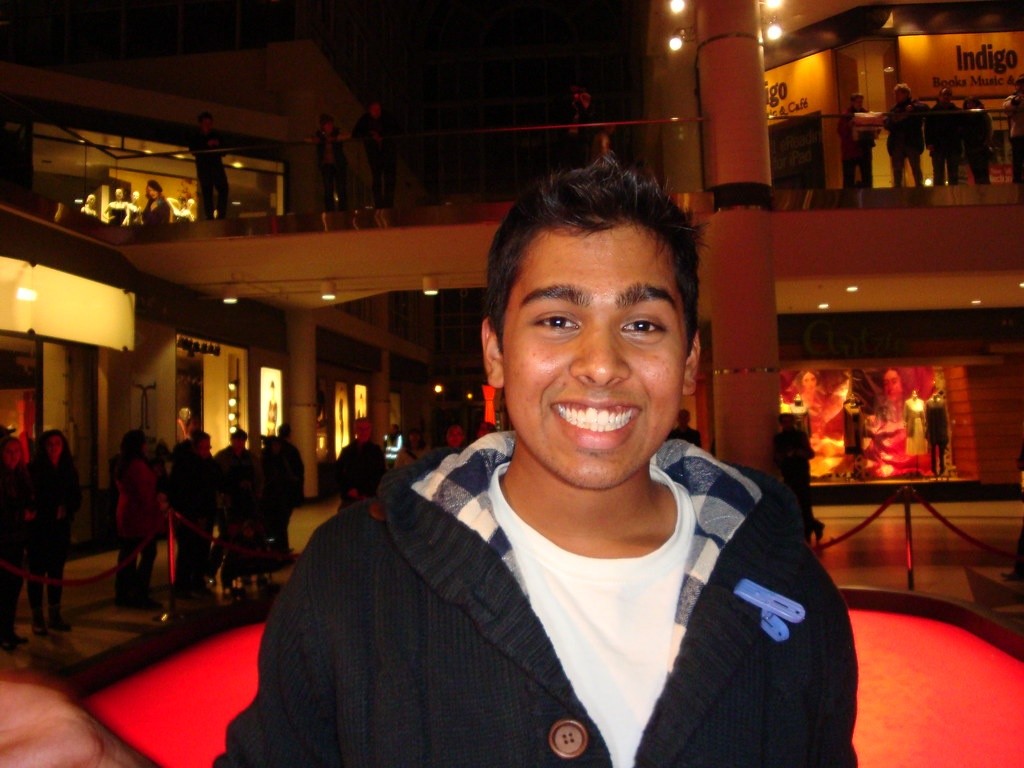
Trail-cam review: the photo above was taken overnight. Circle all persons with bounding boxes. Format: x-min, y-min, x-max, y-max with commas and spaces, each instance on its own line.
167, 192, 197, 222
141, 180, 170, 224
305, 112, 350, 211
109, 421, 305, 610
778, 393, 812, 438
80, 194, 98, 218
334, 415, 500, 510
0, 158, 860, 768
101, 188, 132, 226
187, 111, 228, 220
836, 93, 879, 188
884, 75, 1024, 187
999, 445, 1024, 581
0, 425, 84, 650
551, 84, 616, 164
666, 409, 701, 447
842, 395, 865, 454
903, 391, 927, 455
353, 102, 403, 209
772, 413, 825, 542
924, 392, 949, 477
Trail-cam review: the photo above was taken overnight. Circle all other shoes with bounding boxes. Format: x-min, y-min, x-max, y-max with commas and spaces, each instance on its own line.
0, 632, 29, 651
813, 521, 825, 541
1000, 569, 1024, 581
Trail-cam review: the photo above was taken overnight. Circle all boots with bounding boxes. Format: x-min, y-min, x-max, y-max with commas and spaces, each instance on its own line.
31, 603, 49, 636
47, 603, 70, 631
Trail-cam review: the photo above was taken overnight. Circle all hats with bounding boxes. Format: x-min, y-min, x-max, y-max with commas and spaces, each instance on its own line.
0, 424, 16, 439
121, 428, 149, 450
